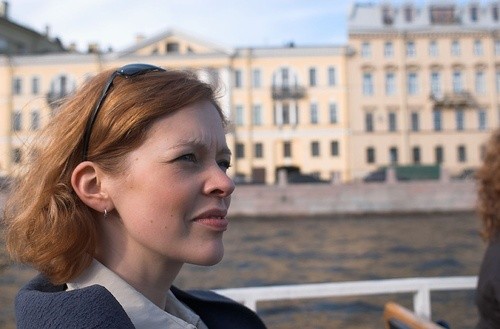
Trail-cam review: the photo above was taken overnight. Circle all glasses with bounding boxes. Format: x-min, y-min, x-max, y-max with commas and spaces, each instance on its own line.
82, 63, 166, 163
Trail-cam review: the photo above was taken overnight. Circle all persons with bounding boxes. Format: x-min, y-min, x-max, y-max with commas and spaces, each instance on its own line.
472, 126, 500, 329
0, 64, 269, 329
